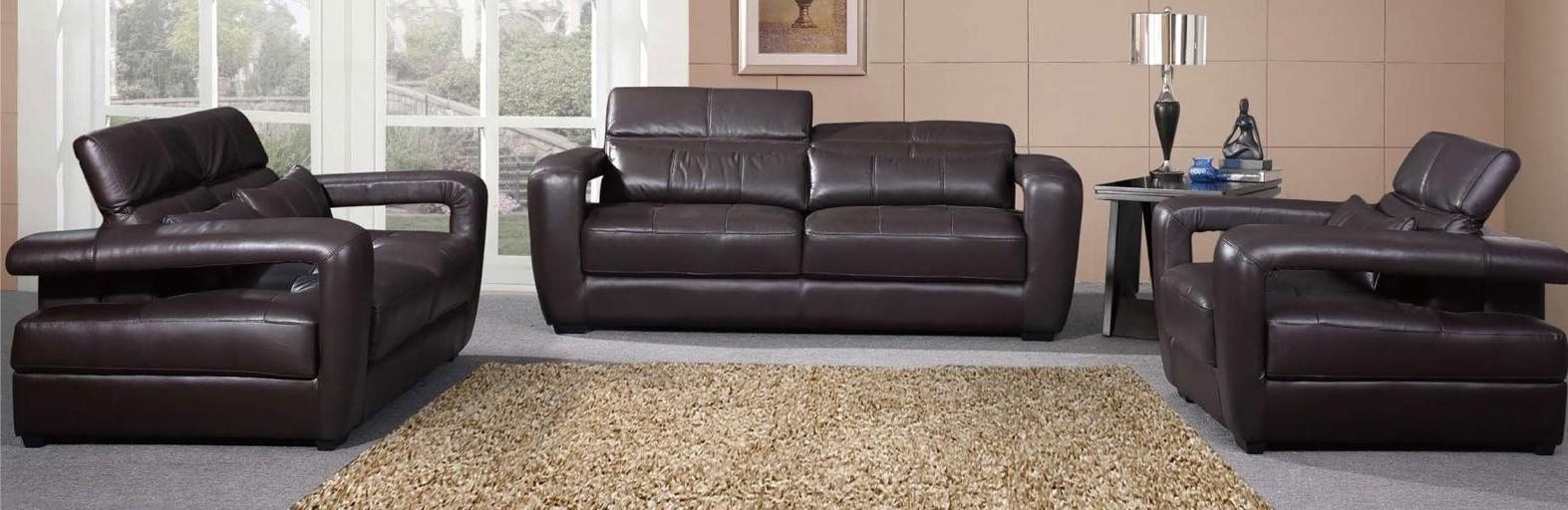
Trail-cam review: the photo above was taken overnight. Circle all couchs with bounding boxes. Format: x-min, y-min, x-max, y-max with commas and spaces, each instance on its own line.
527, 86, 1083, 342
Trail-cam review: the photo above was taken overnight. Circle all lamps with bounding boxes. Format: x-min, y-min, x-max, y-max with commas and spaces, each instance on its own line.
1127, 7, 1209, 183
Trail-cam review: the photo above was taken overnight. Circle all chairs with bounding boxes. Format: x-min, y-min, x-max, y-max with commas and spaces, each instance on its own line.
1146, 130, 1567, 454
4, 105, 486, 452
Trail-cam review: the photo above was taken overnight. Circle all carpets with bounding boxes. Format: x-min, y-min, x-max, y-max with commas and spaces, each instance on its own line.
291, 361, 1276, 510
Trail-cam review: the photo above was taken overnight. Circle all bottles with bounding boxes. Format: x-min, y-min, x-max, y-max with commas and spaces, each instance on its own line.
1190, 157, 1217, 181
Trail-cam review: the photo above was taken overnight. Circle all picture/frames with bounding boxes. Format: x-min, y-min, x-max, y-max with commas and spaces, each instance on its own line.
737, 1, 868, 77
